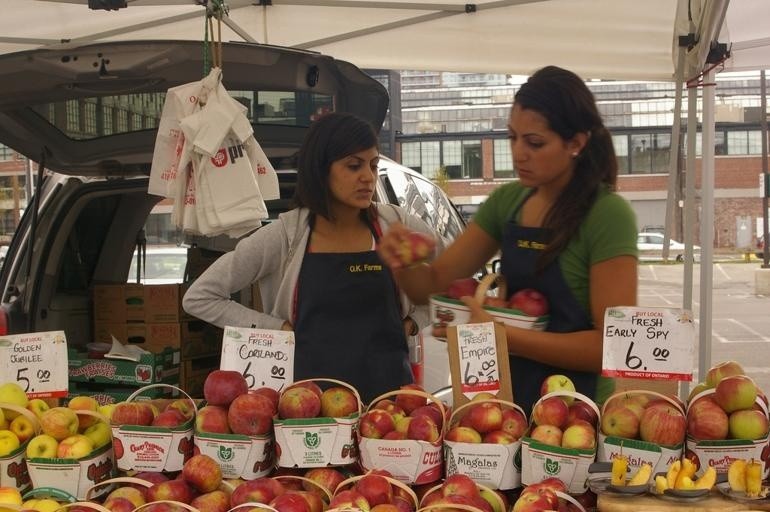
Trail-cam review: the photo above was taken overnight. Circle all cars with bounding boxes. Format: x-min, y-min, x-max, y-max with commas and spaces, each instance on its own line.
755, 232, 770, 260
636, 233, 702, 263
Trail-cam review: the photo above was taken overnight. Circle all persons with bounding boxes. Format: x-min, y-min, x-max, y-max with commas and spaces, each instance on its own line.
181, 110, 432, 412
377, 67, 639, 418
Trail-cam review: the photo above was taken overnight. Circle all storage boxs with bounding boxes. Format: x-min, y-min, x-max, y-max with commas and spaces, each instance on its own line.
93, 323, 222, 359
93, 280, 200, 320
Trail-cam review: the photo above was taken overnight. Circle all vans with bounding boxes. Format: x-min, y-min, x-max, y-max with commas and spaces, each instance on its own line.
1, 39, 503, 402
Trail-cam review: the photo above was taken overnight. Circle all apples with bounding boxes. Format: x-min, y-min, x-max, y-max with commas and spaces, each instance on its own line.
193, 370, 280, 482
27, 397, 118, 501
601, 389, 688, 482
655, 459, 717, 493
110, 384, 202, 473
483, 297, 507, 307
401, 232, 433, 266
509, 289, 548, 317
610, 443, 651, 487
689, 360, 770, 481
727, 459, 761, 495
1, 382, 51, 495
274, 378, 363, 470
447, 277, 478, 300
1, 453, 593, 511
520, 375, 601, 496
443, 392, 528, 491
357, 384, 452, 486
